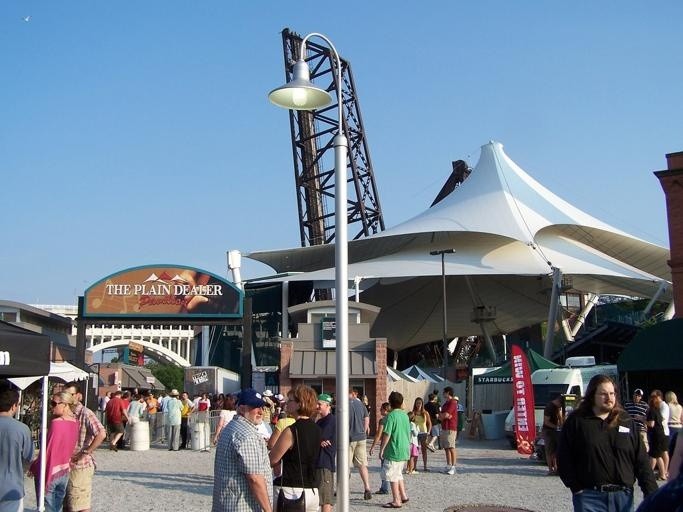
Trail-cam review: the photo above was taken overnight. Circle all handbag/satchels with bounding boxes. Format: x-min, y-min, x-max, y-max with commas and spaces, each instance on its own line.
275, 489, 305, 512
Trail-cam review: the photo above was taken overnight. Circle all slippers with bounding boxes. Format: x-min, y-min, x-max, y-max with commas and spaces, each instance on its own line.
401, 499, 409, 503
381, 503, 402, 509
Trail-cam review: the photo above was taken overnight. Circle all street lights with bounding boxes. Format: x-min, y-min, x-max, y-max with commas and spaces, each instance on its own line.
268, 33, 354, 511
430, 248, 458, 395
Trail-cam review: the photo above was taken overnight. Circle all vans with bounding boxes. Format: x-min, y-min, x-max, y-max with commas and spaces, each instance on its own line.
503, 354, 622, 451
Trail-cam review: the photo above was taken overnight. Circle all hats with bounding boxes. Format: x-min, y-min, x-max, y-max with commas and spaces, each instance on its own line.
454, 396, 459, 401
634, 388, 644, 395
235, 389, 270, 408
263, 389, 273, 396
168, 389, 180, 396
318, 394, 333, 403
274, 393, 284, 400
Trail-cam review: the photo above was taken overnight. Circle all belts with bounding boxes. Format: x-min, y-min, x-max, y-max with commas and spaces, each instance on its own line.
582, 484, 631, 493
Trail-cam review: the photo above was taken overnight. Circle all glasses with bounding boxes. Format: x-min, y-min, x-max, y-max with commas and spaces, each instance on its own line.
50, 400, 65, 407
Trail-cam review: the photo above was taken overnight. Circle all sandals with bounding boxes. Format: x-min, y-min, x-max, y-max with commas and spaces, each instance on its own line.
655, 476, 667, 482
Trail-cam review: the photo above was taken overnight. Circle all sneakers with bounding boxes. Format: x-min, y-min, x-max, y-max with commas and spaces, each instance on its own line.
109, 444, 119, 451
363, 491, 372, 501
405, 470, 420, 475
439, 465, 455, 475
426, 444, 436, 453
375, 491, 386, 494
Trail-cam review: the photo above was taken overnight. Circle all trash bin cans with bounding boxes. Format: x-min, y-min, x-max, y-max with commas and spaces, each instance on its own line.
191, 420, 210, 451
128, 416, 149, 451
